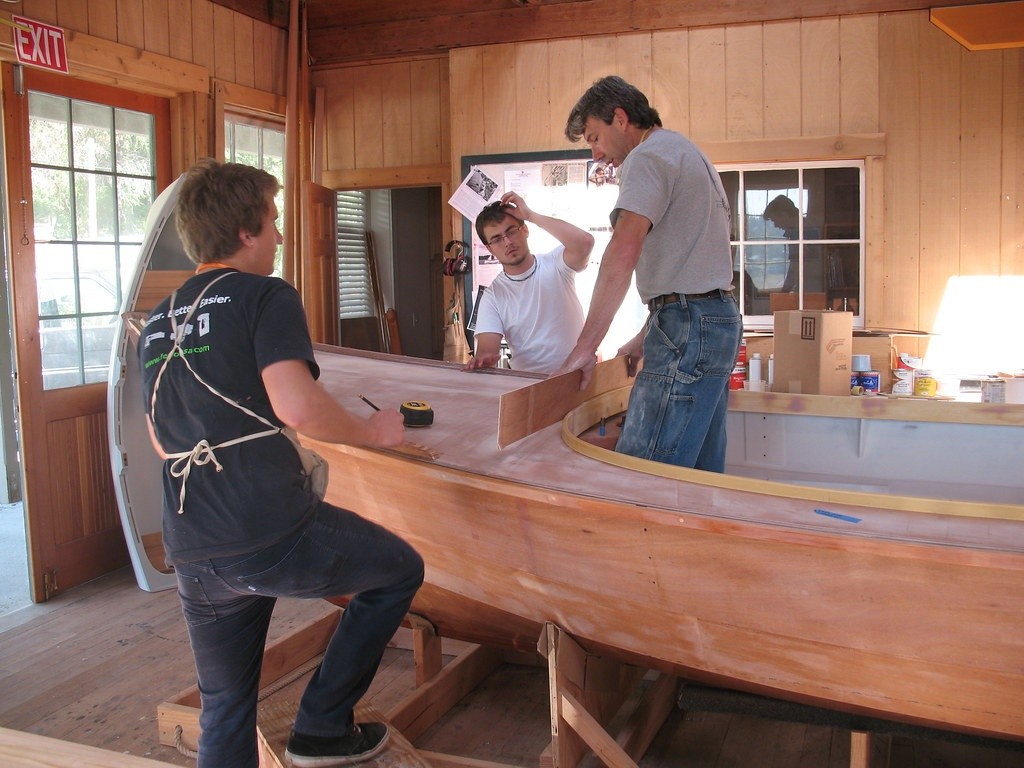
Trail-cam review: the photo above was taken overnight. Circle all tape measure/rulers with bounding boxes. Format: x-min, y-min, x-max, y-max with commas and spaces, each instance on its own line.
399, 401, 435, 428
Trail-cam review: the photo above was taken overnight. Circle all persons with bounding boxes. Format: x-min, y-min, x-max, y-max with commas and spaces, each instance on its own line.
763, 195, 824, 292
136, 159, 425, 768
546, 75, 744, 473
460, 191, 595, 374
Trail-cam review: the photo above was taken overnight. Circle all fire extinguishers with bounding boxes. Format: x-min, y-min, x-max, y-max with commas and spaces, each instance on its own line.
729, 314, 748, 391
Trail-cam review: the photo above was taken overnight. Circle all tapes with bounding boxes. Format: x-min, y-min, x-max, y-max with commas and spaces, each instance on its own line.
852, 386, 864, 395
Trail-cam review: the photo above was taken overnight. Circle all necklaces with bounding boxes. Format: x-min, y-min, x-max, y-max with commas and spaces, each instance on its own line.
503, 254, 537, 282
638, 125, 654, 144
195, 261, 231, 275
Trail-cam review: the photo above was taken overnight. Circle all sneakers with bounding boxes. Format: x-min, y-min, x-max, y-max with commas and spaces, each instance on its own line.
285, 721, 391, 768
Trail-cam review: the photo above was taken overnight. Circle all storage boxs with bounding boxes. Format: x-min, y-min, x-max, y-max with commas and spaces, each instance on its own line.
745, 337, 773, 382
774, 309, 853, 396
851, 330, 927, 393
980, 378, 1024, 404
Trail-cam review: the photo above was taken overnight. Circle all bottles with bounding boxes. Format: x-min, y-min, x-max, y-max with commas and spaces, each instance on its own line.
768, 353, 775, 384
749, 354, 763, 380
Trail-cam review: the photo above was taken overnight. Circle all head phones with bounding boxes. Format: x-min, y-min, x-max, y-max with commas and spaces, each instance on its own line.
442, 240, 469, 277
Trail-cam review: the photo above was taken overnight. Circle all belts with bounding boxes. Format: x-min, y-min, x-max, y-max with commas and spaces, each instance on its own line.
648, 289, 731, 315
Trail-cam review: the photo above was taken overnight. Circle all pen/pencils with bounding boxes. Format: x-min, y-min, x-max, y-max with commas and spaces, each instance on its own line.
357, 394, 381, 411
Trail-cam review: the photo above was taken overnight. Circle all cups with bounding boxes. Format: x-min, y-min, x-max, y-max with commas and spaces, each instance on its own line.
743, 379, 766, 392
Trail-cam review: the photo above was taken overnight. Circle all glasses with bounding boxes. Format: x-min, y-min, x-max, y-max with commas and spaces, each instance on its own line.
487, 224, 528, 248
